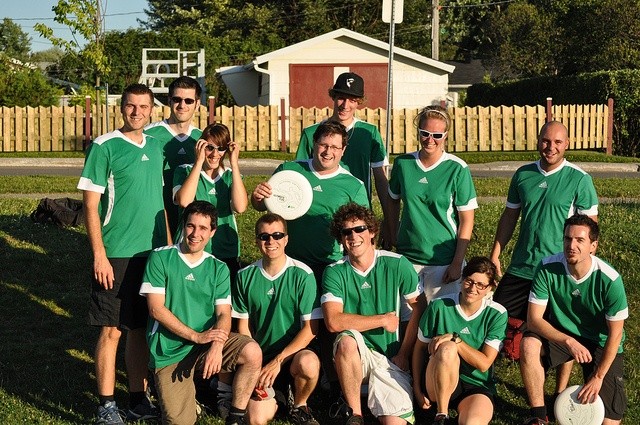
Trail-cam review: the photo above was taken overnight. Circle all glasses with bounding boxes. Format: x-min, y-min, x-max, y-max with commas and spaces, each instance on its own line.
316, 142, 342, 150
171, 97, 196, 104
256, 231, 287, 240
206, 145, 227, 151
341, 225, 369, 235
419, 128, 448, 140
463, 277, 490, 290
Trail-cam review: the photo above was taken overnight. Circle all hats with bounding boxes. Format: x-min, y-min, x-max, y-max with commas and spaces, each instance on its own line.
331, 72, 364, 97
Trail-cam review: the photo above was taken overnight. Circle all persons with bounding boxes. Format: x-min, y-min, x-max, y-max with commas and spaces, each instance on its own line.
387, 105, 480, 322
320, 201, 427, 425
251, 120, 372, 267
231, 213, 322, 425
141, 76, 203, 166
295, 72, 396, 250
172, 122, 249, 259
139, 200, 263, 425
411, 257, 509, 425
518, 214, 629, 425
488, 121, 599, 394
76, 82, 173, 425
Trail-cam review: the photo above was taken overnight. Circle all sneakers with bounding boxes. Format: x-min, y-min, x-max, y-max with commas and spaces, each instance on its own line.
97, 399, 125, 425
289, 404, 320, 425
127, 397, 162, 421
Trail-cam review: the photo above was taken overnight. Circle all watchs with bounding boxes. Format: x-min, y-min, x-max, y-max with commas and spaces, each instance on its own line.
450, 332, 460, 342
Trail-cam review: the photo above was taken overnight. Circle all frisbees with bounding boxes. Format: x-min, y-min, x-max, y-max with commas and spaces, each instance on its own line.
263, 170, 313, 221
554, 385, 606, 424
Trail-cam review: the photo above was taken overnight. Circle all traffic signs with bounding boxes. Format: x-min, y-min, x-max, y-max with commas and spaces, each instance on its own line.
382, 0, 404, 24
